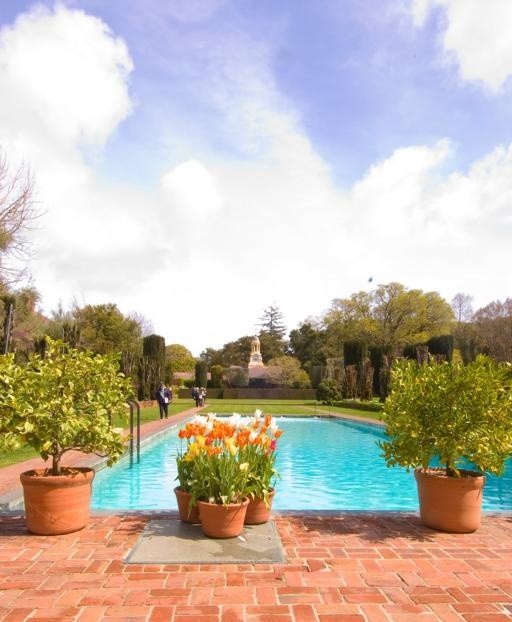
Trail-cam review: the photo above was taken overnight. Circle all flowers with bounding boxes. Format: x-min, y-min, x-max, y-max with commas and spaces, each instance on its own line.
172, 410, 284, 520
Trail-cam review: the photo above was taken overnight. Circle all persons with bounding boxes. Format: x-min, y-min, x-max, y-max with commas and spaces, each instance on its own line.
155, 384, 172, 421
192, 387, 208, 407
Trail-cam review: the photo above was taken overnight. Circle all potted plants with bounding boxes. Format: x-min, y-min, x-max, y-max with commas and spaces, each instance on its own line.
376, 353, 512, 531
0, 333, 135, 536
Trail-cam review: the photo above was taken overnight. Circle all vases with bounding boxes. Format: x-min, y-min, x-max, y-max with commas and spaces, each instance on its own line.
174, 485, 275, 539
